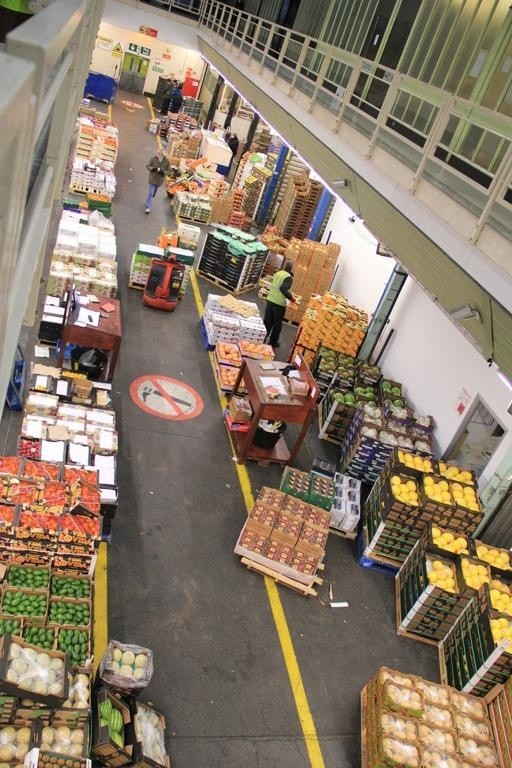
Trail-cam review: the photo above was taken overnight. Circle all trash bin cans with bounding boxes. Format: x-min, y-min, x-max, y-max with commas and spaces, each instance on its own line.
251, 412, 287, 450
71, 347, 108, 381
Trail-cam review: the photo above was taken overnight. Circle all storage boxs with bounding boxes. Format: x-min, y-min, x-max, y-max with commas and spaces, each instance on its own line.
128, 74, 511, 768
0, 74, 121, 768
92, 639, 171, 768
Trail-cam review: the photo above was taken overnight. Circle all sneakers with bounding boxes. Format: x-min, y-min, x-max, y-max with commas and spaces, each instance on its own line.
268, 340, 280, 348
145, 207, 151, 214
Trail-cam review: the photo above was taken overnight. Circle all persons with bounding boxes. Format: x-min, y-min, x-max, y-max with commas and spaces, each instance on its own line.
144, 147, 172, 214
217, 131, 239, 177
264, 261, 296, 349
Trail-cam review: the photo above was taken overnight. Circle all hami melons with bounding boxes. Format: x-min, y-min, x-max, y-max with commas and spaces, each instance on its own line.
0, 642, 148, 768
390, 450, 512, 654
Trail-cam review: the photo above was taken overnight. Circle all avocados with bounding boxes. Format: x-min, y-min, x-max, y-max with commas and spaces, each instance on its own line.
0, 566, 90, 667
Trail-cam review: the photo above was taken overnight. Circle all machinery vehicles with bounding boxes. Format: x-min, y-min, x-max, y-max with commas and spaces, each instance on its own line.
142, 254, 186, 312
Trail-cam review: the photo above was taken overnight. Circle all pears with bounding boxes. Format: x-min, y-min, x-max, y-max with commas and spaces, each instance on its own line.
382, 671, 498, 768
